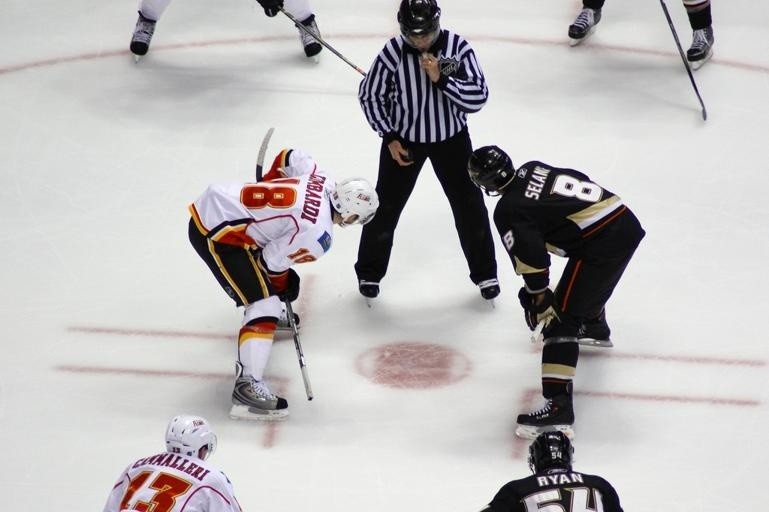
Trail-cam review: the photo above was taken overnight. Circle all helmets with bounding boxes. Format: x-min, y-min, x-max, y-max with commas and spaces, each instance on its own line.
397, 0, 441, 36
328, 177, 380, 226
528, 431, 575, 474
165, 414, 214, 460
466, 144, 518, 191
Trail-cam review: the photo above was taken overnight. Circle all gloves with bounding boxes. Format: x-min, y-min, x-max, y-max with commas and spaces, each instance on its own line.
269, 268, 300, 302
517, 282, 562, 333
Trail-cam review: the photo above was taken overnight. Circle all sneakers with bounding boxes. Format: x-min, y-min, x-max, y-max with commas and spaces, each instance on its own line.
294, 13, 323, 58
568, 5, 601, 39
687, 25, 715, 62
477, 277, 501, 300
516, 389, 575, 427
130, 10, 159, 56
275, 308, 300, 328
575, 318, 611, 342
358, 277, 380, 298
231, 377, 288, 410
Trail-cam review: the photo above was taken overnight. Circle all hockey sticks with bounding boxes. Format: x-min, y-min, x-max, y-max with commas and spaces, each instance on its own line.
255, 127, 314, 401
660, 1, 707, 121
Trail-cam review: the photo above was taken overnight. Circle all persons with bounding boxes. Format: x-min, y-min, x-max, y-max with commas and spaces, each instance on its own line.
478, 431, 624, 511
352, 2, 503, 300
465, 145, 645, 428
101, 413, 243, 512
128, 1, 323, 63
188, 147, 379, 410
563, 1, 721, 63
